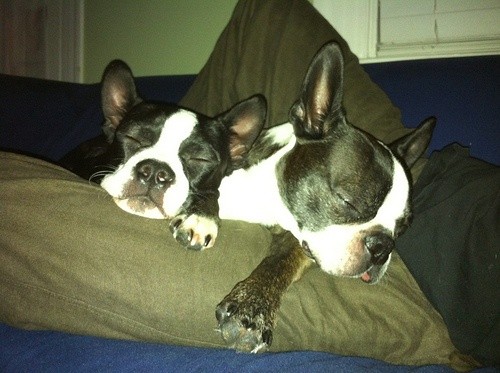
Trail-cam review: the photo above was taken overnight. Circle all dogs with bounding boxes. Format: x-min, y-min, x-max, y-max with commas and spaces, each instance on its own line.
49, 58, 269, 251
214, 39, 439, 354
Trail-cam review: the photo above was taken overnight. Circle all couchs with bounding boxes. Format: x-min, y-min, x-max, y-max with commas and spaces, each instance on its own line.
0, 51, 499, 372
